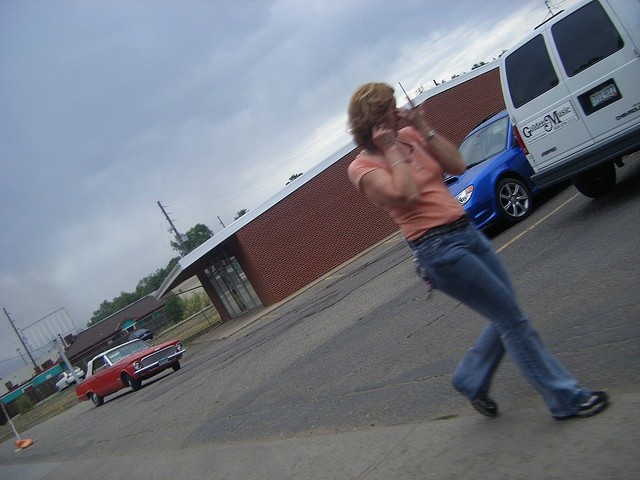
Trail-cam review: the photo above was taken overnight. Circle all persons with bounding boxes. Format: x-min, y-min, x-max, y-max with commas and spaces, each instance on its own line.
347, 82, 609, 420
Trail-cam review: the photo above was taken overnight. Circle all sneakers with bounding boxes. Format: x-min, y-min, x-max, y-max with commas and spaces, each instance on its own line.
551, 392, 607, 420
452, 377, 497, 419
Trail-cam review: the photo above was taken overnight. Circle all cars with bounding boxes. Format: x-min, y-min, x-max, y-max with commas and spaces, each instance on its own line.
128, 328, 154, 341
443, 108, 540, 233
75, 337, 187, 407
55, 366, 84, 392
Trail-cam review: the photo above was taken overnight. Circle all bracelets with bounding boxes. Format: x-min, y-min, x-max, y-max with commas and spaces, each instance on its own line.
425, 129, 436, 140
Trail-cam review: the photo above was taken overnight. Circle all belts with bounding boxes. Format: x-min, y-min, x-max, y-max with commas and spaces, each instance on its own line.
405, 214, 468, 247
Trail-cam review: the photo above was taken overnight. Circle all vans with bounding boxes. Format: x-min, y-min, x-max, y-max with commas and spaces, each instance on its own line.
498, 1, 640, 198
